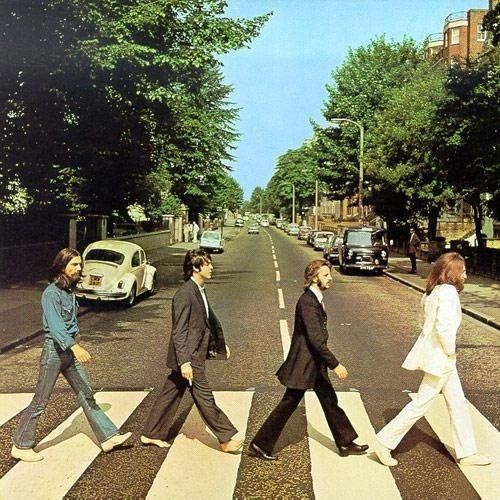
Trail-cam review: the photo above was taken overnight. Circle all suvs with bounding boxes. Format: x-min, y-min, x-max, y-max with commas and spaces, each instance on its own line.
338, 228, 395, 276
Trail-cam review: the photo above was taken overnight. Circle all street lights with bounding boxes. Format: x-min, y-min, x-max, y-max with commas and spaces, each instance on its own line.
279, 182, 296, 223
331, 118, 364, 225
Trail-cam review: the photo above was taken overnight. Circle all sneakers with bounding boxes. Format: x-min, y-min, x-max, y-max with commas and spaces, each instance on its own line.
459, 454, 494, 465
11, 444, 44, 462
100, 432, 132, 452
369, 436, 398, 466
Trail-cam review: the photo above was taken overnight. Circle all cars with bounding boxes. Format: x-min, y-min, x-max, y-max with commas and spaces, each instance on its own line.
200, 230, 225, 253
72, 240, 158, 307
235, 210, 340, 264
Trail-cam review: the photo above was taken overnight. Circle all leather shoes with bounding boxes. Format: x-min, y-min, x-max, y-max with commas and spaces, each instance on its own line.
338, 442, 369, 456
248, 441, 278, 461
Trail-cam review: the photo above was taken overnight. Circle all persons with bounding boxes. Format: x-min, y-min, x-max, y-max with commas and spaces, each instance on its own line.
250, 258, 369, 460
139, 249, 247, 453
369, 252, 493, 466
8, 247, 133, 462
178, 210, 229, 243
405, 227, 420, 273
